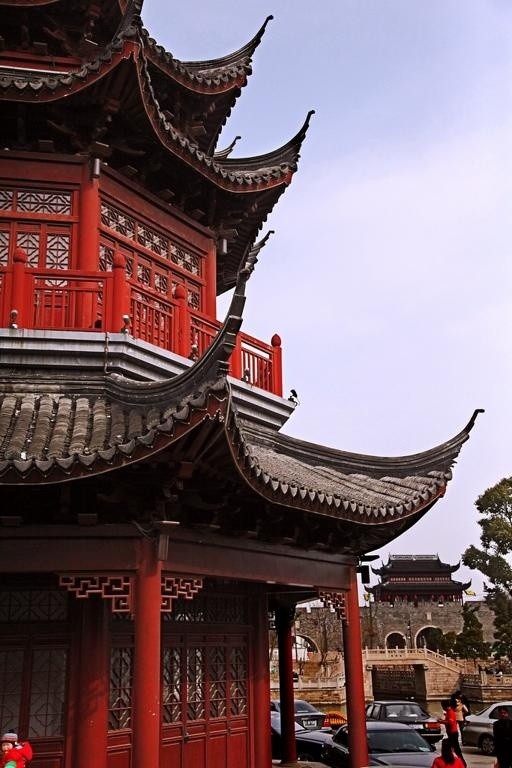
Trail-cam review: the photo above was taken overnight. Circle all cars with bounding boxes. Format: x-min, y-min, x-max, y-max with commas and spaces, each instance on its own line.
460, 699, 512, 755
270, 698, 445, 767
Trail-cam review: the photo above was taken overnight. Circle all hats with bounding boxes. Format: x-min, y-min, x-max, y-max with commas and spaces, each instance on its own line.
0, 733, 19, 746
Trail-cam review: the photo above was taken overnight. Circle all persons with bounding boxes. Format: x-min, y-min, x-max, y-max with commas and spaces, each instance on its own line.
0, 729, 33, 768
450, 694, 468, 747
495, 669, 503, 682
492, 705, 512, 768
271, 665, 276, 673
437, 698, 468, 768
456, 690, 471, 724
293, 668, 299, 689
431, 738, 466, 768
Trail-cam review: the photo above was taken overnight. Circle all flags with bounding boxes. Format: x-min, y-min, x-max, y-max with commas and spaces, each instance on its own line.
464, 589, 476, 597
363, 593, 370, 601
